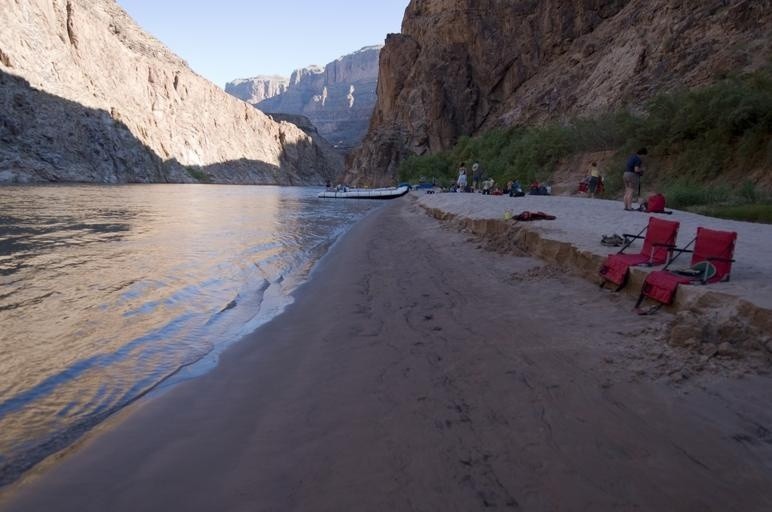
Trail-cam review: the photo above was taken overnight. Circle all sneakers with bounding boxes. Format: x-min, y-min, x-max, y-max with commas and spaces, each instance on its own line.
624, 207, 634, 211
601, 234, 630, 246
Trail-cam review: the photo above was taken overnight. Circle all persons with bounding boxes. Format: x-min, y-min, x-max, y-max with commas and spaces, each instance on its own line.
530, 177, 539, 192
586, 162, 599, 198
482, 176, 514, 195
623, 148, 647, 210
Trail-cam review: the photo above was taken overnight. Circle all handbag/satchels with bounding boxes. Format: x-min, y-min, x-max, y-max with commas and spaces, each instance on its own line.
648, 193, 665, 211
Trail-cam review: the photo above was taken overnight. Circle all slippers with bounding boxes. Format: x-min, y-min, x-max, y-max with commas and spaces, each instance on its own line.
513, 210, 556, 221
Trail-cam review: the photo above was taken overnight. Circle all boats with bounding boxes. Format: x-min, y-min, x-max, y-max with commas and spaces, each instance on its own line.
318, 185, 409, 198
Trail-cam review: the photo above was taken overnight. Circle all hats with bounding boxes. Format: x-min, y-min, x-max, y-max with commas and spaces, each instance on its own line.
472, 163, 479, 171
511, 176, 517, 180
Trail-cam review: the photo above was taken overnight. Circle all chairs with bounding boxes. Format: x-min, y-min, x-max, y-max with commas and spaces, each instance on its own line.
598, 216, 737, 308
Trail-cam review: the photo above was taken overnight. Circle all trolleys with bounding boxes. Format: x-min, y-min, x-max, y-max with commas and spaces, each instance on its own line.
635, 170, 672, 215
